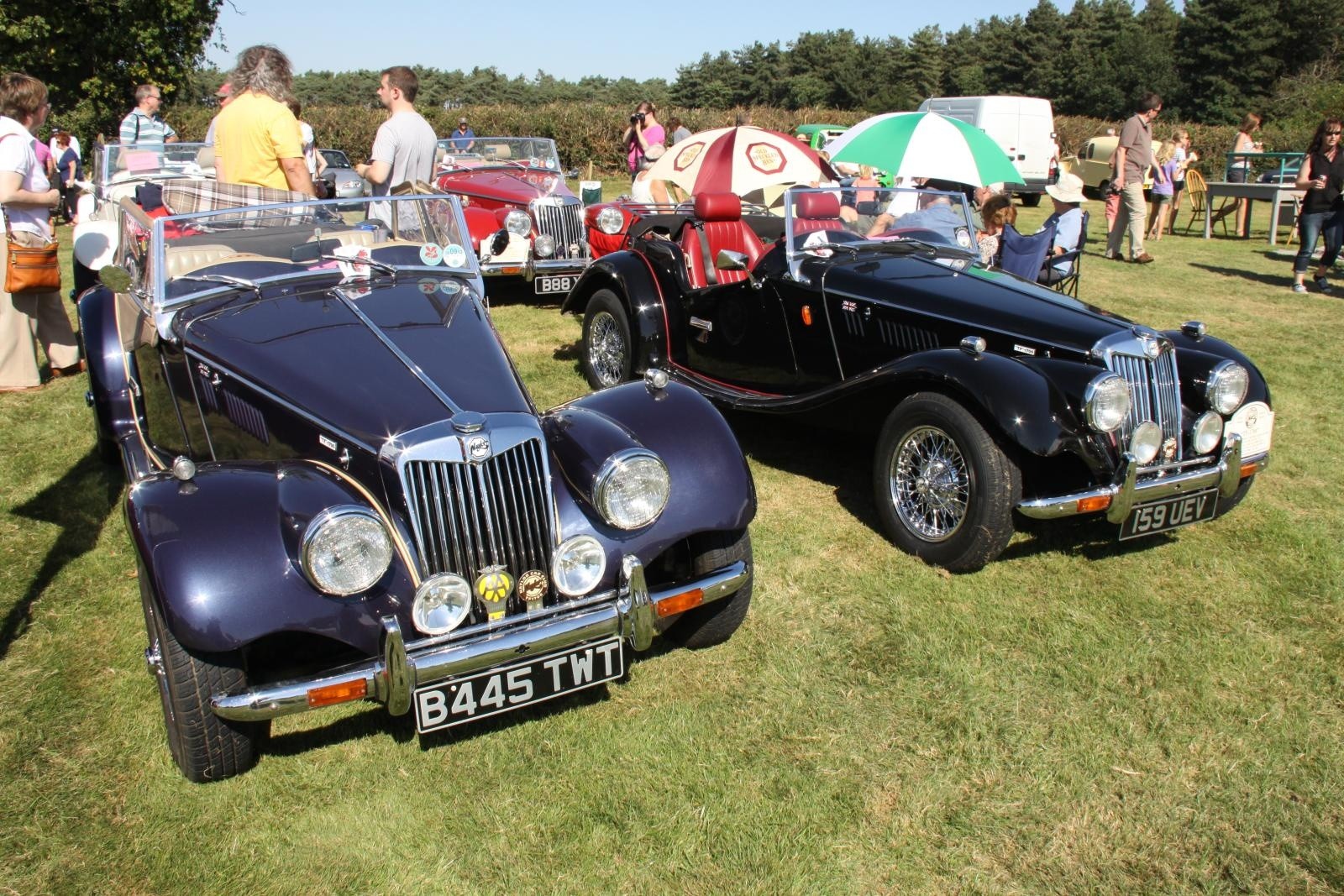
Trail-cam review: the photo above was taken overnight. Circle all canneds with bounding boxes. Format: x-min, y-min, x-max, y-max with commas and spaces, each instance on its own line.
1189, 152, 1197, 162
1319, 175, 1327, 188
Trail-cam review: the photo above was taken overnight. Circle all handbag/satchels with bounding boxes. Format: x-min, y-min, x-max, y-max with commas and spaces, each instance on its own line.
4, 242, 62, 293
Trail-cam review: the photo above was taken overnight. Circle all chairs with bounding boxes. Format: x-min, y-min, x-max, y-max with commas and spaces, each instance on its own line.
1185, 169, 1229, 236
791, 191, 844, 236
1042, 209, 1090, 297
166, 245, 237, 281
196, 147, 215, 171
995, 213, 1059, 287
309, 230, 373, 265
482, 144, 511, 167
112, 149, 150, 179
1287, 193, 1305, 246
681, 192, 764, 289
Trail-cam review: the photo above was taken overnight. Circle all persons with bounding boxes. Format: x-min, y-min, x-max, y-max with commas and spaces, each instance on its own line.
666, 117, 694, 203
1210, 114, 1264, 239
623, 101, 665, 181
450, 117, 476, 154
119, 84, 178, 169
282, 96, 327, 180
1291, 115, 1344, 294
1035, 174, 1087, 284
1106, 92, 1166, 263
632, 146, 676, 215
1145, 126, 1193, 242
825, 153, 1018, 265
213, 84, 234, 108
355, 67, 439, 243
0, 72, 81, 394
740, 103, 754, 124
214, 47, 314, 195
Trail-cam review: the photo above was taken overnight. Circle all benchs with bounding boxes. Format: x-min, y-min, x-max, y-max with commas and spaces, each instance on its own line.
164, 224, 353, 258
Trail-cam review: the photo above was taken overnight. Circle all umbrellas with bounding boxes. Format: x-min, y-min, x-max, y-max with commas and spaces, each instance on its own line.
642, 112, 838, 200
821, 93, 1027, 211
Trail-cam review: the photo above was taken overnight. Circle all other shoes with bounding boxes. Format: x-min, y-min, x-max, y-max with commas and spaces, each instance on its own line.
0, 385, 44, 395
65, 220, 76, 226
1209, 217, 1215, 236
51, 359, 87, 378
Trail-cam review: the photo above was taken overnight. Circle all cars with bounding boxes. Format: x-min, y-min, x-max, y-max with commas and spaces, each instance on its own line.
77, 196, 756, 785
429, 138, 593, 296
92, 143, 363, 213
1059, 136, 1163, 202
561, 191, 1274, 574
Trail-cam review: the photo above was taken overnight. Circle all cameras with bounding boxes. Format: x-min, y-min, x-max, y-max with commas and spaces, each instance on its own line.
1104, 183, 1124, 196
630, 113, 645, 125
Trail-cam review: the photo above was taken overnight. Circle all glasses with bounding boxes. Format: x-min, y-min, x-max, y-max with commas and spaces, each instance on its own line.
149, 96, 162, 100
57, 141, 61, 144
1183, 137, 1189, 140
1153, 109, 1160, 114
1326, 131, 1341, 135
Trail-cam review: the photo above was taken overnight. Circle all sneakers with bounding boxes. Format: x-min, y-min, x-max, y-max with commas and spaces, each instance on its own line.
1111, 253, 1124, 260
1292, 283, 1307, 292
1134, 253, 1154, 264
1314, 274, 1332, 293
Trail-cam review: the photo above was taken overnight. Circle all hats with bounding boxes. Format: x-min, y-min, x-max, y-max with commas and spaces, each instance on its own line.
213, 84, 232, 97
459, 117, 467, 123
912, 178, 954, 192
1045, 173, 1088, 202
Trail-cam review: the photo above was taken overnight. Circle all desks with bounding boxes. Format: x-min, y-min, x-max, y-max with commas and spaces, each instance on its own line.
1224, 151, 1305, 184
1204, 181, 1307, 245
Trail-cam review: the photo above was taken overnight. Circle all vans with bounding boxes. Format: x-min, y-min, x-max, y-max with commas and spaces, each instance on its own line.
918, 96, 1059, 207
